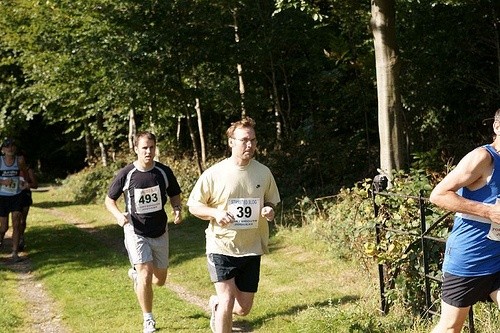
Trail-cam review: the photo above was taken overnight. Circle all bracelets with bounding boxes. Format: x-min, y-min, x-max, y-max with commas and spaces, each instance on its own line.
264, 202, 277, 213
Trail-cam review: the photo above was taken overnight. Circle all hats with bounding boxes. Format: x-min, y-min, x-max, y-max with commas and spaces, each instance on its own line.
3, 139, 15, 145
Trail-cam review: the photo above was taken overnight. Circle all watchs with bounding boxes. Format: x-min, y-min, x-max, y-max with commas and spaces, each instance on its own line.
173, 206, 182, 209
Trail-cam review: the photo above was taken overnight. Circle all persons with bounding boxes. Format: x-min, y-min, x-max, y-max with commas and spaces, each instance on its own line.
186, 116, 280, 333
429, 108, 500, 333
0, 138, 38, 262
105, 132, 181, 333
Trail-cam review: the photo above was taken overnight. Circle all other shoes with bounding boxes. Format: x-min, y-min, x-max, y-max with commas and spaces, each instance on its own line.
12, 254, 20, 263
18, 238, 23, 250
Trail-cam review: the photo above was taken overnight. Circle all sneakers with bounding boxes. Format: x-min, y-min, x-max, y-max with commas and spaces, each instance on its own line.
210, 296, 220, 332
143, 319, 156, 333
128, 268, 138, 294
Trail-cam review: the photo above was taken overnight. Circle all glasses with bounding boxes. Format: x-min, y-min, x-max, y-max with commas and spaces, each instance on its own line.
232, 136, 258, 146
